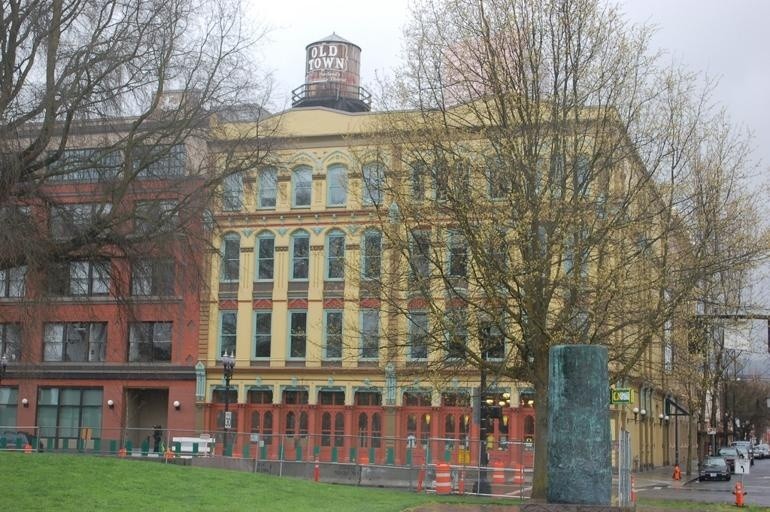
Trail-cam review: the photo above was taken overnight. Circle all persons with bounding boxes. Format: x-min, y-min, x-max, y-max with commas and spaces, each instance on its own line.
151, 426, 161, 452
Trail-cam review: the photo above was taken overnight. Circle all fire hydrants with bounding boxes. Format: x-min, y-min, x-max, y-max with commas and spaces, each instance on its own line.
153, 424, 162, 452
672, 466, 682, 480
732, 482, 748, 507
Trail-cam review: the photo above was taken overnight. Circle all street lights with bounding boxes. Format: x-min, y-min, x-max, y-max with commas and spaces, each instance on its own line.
223, 349, 236, 456
473, 314, 492, 494
0, 356, 8, 378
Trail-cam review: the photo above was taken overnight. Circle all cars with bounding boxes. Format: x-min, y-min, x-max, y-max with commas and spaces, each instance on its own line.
0, 431, 44, 452
699, 440, 770, 481
